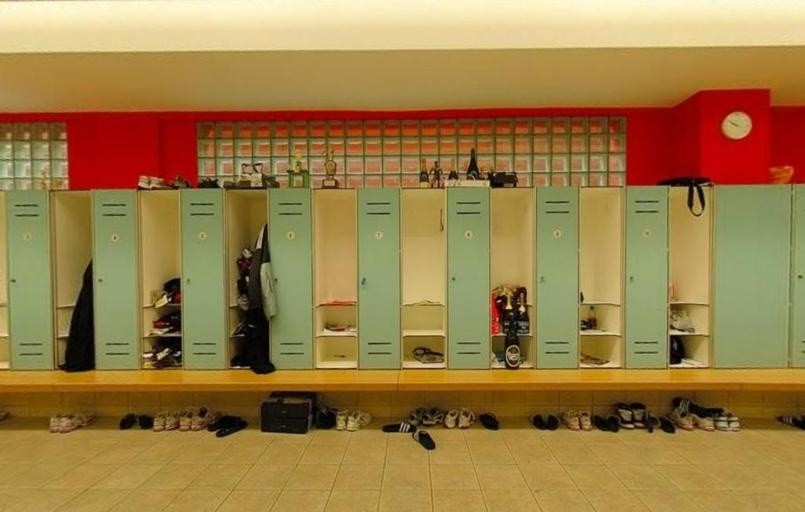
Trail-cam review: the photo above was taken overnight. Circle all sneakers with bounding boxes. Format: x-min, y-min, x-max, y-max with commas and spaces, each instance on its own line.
408, 404, 500, 429
528, 396, 741, 434
137, 174, 221, 190
118, 404, 249, 438
49, 413, 97, 433
316, 406, 373, 431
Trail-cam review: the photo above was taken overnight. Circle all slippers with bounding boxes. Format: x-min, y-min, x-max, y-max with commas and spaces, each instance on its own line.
777, 414, 805, 430
382, 420, 416, 433
412, 429, 435, 450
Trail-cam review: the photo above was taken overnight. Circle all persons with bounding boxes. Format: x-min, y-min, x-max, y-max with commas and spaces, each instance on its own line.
429, 160, 443, 187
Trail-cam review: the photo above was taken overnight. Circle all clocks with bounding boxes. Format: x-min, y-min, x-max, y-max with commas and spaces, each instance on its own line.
721, 110, 752, 140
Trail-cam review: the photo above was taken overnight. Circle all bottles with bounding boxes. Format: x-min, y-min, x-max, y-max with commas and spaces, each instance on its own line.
449, 158, 458, 180
419, 158, 429, 188
503, 318, 521, 369
588, 305, 597, 329
465, 148, 480, 180
501, 291, 529, 333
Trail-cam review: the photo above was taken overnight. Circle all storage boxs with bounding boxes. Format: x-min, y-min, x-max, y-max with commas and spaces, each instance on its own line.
260, 414, 314, 433
262, 391, 316, 417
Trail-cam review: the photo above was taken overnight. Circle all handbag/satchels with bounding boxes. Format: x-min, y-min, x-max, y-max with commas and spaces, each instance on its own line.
656, 176, 710, 187
487, 171, 518, 188
